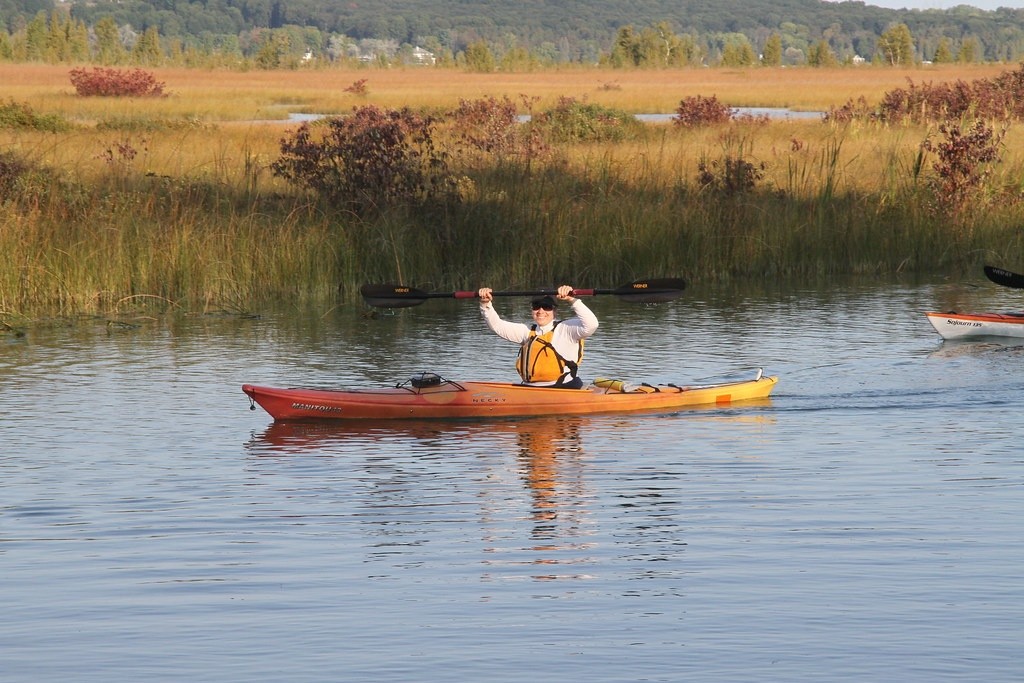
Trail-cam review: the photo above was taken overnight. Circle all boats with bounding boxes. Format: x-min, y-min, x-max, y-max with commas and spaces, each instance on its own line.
924, 309, 1024, 340
242, 368, 778, 420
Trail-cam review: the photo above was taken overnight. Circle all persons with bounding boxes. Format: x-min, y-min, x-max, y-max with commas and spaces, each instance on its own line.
479, 285, 599, 390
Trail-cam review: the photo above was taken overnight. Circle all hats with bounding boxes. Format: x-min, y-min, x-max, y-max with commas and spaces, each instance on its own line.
529, 289, 560, 306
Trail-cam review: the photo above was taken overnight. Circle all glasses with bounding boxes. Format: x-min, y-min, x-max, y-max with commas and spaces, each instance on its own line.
530, 303, 554, 311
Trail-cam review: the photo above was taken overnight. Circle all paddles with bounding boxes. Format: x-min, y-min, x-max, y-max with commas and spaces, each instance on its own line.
361, 278, 686, 309
983, 265, 1024, 289
